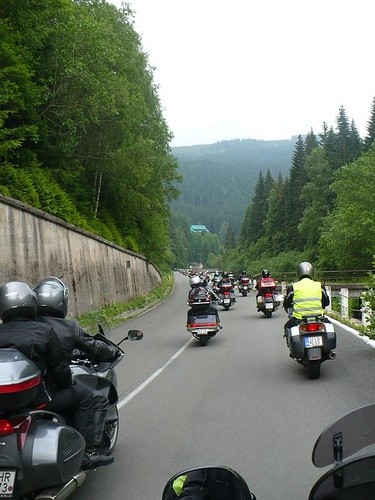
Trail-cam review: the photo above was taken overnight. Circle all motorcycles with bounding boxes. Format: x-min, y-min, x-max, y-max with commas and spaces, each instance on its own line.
0, 325, 144, 500
202, 273, 238, 297
185, 294, 223, 347
252, 279, 281, 318
280, 284, 337, 379
215, 282, 237, 312
237, 277, 253, 298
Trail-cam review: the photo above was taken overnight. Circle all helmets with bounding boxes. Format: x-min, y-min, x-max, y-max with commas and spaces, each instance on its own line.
32, 276, 69, 318
242, 270, 246, 275
262, 269, 270, 277
201, 280, 207, 287
297, 262, 314, 280
0, 281, 38, 323
222, 271, 229, 278
189, 276, 202, 288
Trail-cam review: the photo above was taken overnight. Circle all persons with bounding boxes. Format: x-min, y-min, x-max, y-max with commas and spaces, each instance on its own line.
180, 269, 279, 329
283, 262, 330, 358
0, 277, 121, 470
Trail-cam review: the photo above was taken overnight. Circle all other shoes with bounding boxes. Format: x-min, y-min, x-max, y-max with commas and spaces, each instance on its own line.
80, 450, 115, 471
